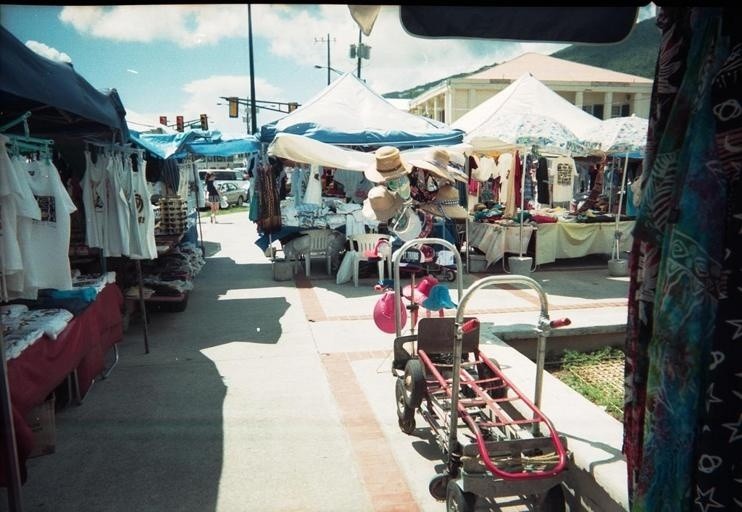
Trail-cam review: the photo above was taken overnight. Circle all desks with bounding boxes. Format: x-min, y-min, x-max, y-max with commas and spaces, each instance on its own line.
468, 216, 634, 274
0, 278, 129, 489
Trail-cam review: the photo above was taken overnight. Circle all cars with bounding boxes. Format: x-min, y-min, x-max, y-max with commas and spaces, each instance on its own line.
226, 160, 249, 180
198, 181, 248, 209
242, 167, 291, 198
197, 169, 251, 202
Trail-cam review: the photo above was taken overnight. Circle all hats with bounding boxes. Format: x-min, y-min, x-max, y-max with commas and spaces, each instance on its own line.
470, 202, 505, 220
365, 146, 468, 333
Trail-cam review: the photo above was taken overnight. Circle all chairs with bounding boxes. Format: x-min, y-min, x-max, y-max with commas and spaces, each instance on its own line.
299, 229, 335, 279
347, 231, 395, 288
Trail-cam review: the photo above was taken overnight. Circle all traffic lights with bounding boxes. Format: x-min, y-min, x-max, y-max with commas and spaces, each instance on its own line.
176, 115, 184, 133
160, 116, 167, 127
200, 115, 207, 131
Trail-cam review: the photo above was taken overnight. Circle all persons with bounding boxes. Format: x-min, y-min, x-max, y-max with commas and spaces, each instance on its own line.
204, 171, 224, 223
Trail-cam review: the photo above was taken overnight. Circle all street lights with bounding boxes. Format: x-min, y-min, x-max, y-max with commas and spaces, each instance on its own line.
216, 97, 249, 134
315, 66, 367, 89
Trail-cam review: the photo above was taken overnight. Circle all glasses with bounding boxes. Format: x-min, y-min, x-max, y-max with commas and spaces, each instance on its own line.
159, 199, 188, 234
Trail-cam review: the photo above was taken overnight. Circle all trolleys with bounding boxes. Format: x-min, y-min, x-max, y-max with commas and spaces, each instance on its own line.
391, 237, 571, 512
429, 216, 458, 282
